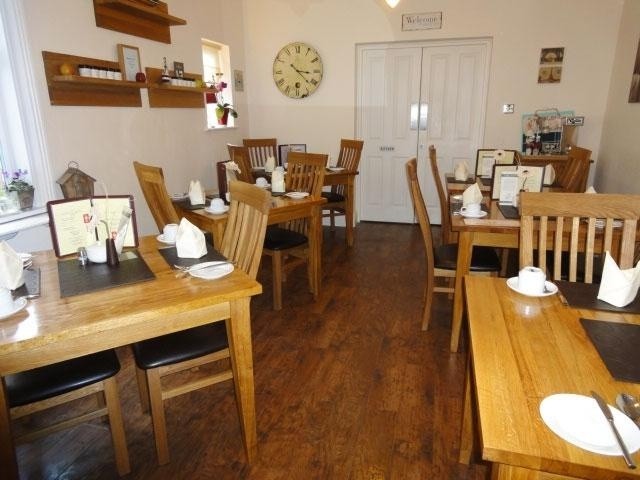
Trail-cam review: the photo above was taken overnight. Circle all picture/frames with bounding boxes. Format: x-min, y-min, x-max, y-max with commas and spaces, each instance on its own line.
234, 70, 244, 92
117, 43, 142, 83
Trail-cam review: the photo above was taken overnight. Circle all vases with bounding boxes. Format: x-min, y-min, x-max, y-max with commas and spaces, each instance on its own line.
217, 107, 230, 125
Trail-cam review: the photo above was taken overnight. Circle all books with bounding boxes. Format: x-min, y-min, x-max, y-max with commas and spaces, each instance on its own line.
279, 145, 306, 169
47, 195, 139, 258
475, 149, 515, 179
215, 160, 237, 200
491, 165, 546, 202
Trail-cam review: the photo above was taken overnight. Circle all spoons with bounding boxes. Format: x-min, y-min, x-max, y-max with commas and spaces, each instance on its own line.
615, 391, 640, 428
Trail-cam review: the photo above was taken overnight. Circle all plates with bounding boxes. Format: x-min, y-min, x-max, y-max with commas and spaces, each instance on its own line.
458, 211, 488, 220
204, 206, 230, 215
16, 249, 33, 269
1, 297, 27, 320
188, 260, 236, 281
285, 192, 310, 200
157, 233, 179, 245
539, 392, 640, 462
506, 276, 560, 298
328, 167, 346, 173
253, 184, 271, 190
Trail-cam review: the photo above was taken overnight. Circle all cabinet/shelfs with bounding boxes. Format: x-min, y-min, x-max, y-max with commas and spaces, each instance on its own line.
39, 0, 220, 109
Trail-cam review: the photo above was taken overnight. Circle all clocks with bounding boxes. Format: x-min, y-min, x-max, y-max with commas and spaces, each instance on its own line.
272, 41, 325, 100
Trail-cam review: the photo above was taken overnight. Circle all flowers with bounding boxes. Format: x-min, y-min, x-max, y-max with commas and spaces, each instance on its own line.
215, 80, 239, 120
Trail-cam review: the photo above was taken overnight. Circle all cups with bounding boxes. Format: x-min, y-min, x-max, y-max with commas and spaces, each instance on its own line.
1, 285, 15, 313
162, 223, 180, 241
518, 266, 545, 295
210, 198, 226, 211
460, 204, 481, 216
284, 163, 289, 170
256, 177, 267, 187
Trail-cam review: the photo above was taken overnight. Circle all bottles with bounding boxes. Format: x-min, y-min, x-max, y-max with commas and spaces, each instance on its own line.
77, 246, 90, 267
525, 135, 542, 156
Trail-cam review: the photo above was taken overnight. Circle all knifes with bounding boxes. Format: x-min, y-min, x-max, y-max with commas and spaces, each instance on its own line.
590, 387, 636, 471
